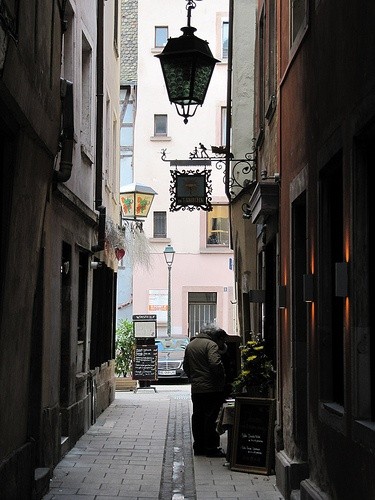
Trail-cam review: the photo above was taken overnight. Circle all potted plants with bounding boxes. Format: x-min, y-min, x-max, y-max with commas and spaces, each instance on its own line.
115, 318, 138, 391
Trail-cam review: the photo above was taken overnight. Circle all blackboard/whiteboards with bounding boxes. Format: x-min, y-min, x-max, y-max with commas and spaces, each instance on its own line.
133, 345, 158, 381
231, 397, 277, 475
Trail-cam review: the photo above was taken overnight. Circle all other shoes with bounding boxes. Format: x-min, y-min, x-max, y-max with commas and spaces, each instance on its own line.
194, 448, 226, 457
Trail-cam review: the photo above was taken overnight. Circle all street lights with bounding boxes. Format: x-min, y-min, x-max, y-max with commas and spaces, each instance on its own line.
163, 244, 175, 336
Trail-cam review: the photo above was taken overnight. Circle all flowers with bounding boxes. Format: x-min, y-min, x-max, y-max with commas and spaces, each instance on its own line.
232, 340, 276, 397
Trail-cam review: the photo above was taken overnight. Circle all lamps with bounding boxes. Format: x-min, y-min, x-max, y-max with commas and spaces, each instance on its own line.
154, 0, 222, 124
120, 185, 159, 231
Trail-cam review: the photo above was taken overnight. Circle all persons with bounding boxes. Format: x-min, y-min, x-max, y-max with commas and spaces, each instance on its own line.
183, 324, 237, 457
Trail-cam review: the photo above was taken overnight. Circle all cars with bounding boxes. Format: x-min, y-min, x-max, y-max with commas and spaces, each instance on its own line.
155, 335, 190, 384
